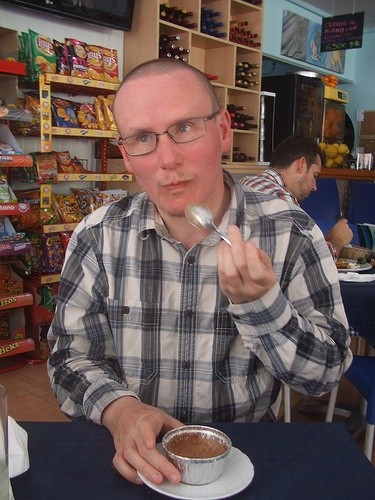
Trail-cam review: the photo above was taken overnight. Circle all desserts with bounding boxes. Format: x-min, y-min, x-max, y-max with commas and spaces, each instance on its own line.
161, 424, 233, 485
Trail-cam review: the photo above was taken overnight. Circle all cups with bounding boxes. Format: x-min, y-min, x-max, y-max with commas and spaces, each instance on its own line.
161, 425, 231, 486
0, 383, 10, 500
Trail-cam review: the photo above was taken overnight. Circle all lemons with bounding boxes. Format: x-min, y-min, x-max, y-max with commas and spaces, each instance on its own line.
318, 142, 350, 168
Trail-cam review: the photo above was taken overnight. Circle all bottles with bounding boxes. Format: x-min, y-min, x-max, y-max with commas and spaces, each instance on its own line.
230, 20, 261, 48
227, 104, 258, 130
158, 34, 190, 64
159, 3, 198, 30
205, 73, 219, 80
236, 62, 260, 88
233, 147, 255, 163
200, 6, 227, 39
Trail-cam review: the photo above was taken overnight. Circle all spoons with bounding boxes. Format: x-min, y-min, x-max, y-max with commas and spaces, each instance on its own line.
184, 203, 231, 246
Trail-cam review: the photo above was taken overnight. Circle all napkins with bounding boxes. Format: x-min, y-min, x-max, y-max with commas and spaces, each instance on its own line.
8, 415, 30, 478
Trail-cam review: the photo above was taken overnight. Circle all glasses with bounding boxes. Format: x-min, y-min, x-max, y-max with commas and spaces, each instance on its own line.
116, 110, 220, 156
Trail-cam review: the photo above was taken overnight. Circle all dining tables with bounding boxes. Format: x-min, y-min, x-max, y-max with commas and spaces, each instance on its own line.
293, 266, 375, 440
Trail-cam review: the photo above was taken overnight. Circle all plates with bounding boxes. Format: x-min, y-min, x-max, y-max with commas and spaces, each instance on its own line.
137, 442, 255, 500
337, 262, 373, 272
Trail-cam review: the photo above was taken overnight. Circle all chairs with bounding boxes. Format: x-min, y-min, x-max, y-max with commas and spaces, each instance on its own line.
325, 354, 375, 463
299, 178, 341, 236
344, 180, 375, 247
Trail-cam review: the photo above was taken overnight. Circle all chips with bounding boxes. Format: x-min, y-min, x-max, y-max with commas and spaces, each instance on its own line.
34, 56, 57, 74
89, 69, 120, 84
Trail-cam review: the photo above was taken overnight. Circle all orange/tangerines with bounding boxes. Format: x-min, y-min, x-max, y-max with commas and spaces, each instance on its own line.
323, 75, 338, 87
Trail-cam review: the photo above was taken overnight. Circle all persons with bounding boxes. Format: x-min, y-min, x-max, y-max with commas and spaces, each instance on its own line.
236, 135, 354, 263
45, 58, 353, 487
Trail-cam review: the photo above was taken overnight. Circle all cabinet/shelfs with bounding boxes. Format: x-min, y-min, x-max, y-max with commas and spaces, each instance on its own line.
0, 58, 37, 373
123, 0, 265, 166
10, 72, 133, 364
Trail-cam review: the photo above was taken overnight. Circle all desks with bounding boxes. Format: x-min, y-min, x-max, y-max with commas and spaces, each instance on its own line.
10, 421, 375, 500
